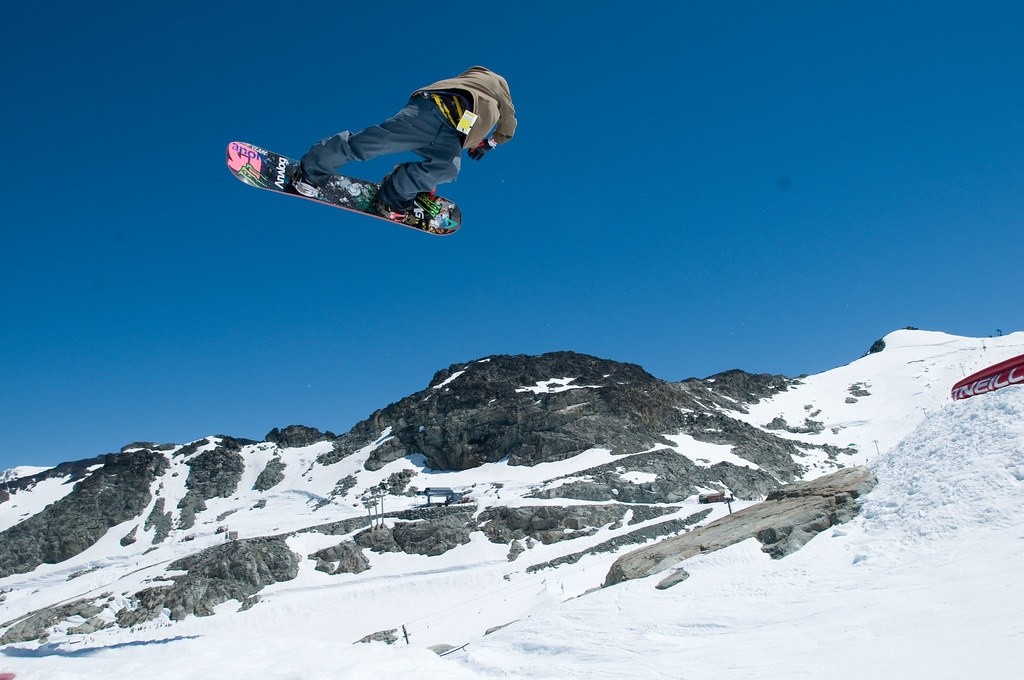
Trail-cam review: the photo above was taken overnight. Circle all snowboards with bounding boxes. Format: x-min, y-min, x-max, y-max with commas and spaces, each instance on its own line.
225, 140, 462, 236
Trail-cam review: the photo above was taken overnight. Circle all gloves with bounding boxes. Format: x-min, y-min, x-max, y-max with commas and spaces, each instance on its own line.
468, 137, 497, 161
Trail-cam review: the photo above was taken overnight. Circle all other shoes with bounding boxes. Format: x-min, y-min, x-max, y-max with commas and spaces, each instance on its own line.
379, 200, 406, 222
292, 165, 321, 197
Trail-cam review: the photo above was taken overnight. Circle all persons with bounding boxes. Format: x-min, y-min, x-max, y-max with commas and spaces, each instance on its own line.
293, 66, 517, 220
